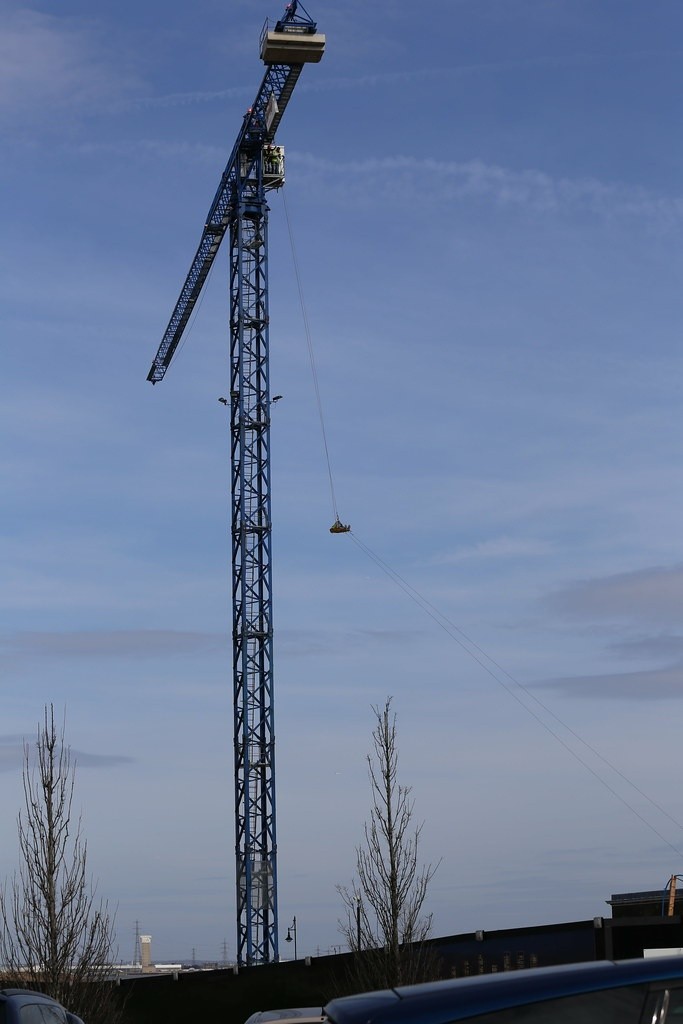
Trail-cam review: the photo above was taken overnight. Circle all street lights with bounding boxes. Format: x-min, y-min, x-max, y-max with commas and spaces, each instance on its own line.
284, 928, 296, 961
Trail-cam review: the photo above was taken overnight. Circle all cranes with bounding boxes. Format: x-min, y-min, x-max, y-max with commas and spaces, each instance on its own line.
143, 2, 328, 967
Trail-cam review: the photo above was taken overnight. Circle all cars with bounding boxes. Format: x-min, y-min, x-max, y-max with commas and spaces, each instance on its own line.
244, 1006, 324, 1024
320, 954, 683, 1024
0, 989, 87, 1024
